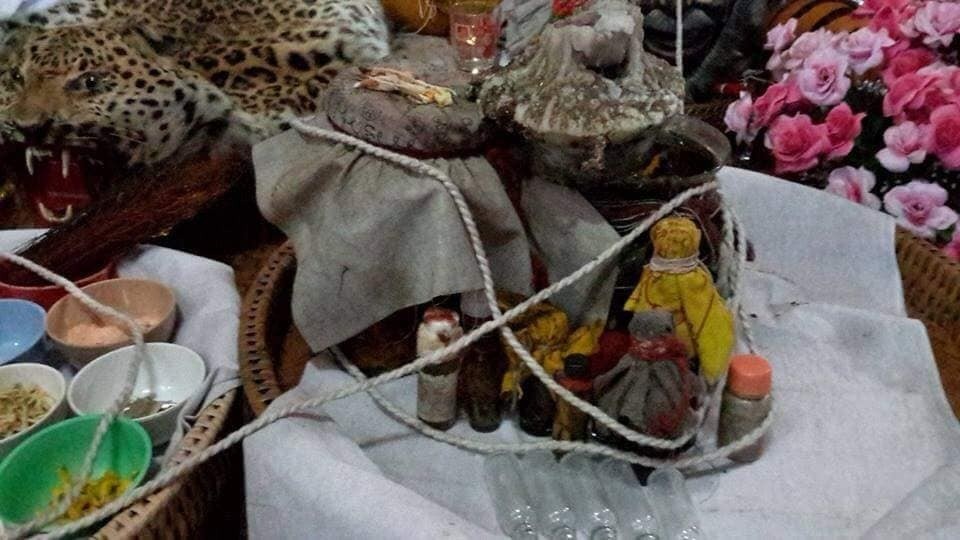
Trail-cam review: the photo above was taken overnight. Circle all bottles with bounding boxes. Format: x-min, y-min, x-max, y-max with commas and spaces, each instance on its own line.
410, 216, 772, 540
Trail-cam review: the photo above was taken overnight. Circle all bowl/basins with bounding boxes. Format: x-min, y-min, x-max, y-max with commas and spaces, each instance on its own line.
0, 262, 206, 533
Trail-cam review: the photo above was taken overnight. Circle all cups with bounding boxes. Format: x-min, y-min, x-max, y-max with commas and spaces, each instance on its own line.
450, 3, 501, 73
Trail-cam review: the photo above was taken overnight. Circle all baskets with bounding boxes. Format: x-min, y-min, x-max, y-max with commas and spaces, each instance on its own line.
237, 164, 958, 538
0, 228, 243, 540
684, 98, 732, 145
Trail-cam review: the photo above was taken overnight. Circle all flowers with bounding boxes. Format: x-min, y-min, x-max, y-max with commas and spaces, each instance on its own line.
721, 0, 960, 264
